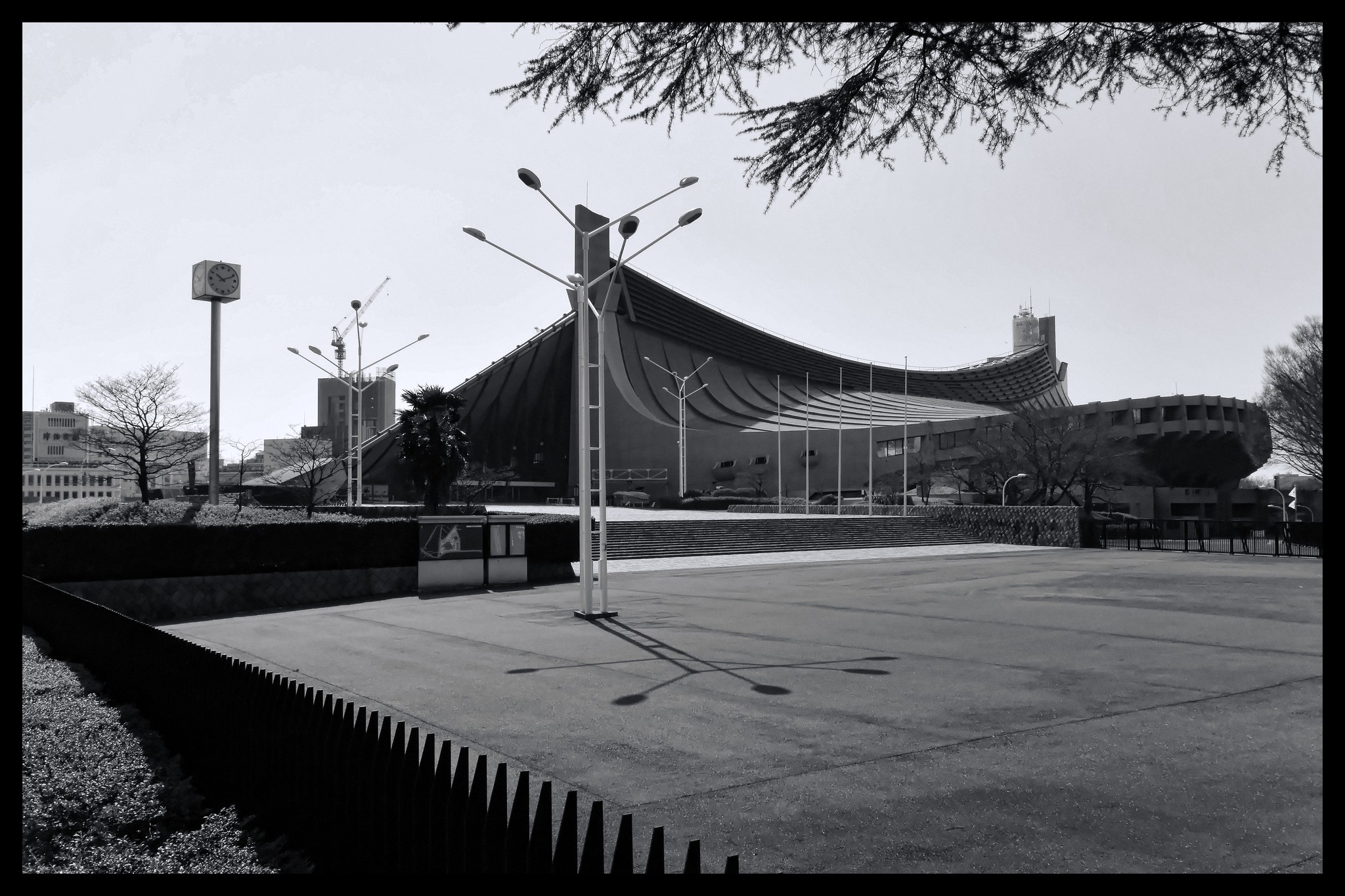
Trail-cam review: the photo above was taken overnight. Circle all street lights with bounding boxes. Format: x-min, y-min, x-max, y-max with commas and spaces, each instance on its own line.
1296, 504, 1314, 521
455, 166, 702, 617
40, 462, 69, 505
1261, 485, 1285, 522
289, 299, 431, 505
1002, 473, 1026, 506
643, 350, 712, 495
1267, 503, 1289, 543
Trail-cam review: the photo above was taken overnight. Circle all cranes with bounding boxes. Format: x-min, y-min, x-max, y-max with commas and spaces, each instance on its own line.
331, 275, 391, 378
